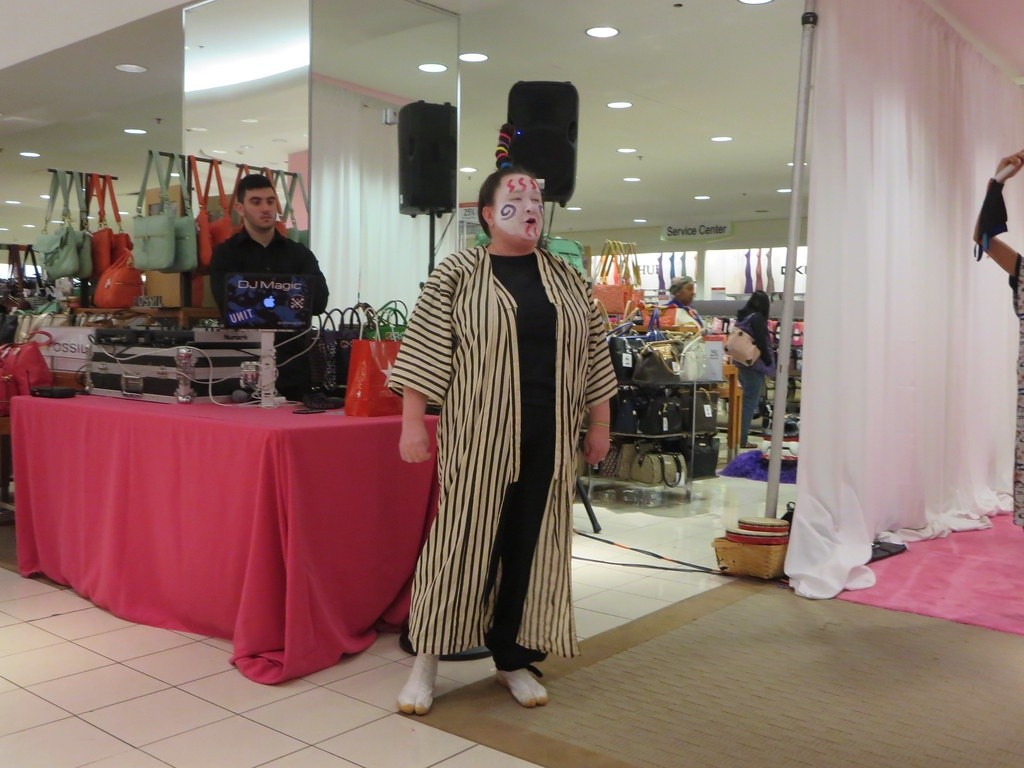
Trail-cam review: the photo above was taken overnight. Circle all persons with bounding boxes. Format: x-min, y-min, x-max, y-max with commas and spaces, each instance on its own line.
209, 175, 328, 328
973, 155, 1024, 526
665, 276, 703, 327
389, 123, 620, 714
730, 290, 772, 448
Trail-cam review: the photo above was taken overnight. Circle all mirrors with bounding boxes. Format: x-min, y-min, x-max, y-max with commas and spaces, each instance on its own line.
183, 0, 461, 331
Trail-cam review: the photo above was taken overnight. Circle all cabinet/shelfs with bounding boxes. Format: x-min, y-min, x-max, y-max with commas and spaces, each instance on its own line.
579, 316, 804, 502
0, 291, 226, 512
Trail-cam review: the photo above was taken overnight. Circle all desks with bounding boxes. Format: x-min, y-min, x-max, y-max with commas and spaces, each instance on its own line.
12, 394, 445, 639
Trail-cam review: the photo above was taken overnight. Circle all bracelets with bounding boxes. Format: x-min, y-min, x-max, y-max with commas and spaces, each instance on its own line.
592, 422, 610, 427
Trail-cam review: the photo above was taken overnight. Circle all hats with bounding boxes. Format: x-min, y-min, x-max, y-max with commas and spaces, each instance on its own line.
668, 276, 692, 294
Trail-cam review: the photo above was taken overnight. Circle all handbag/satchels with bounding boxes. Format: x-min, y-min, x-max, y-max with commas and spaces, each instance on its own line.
0, 244, 72, 343
726, 313, 763, 367
132, 150, 313, 274
31, 169, 145, 308
590, 240, 647, 314
591, 297, 725, 486
545, 236, 586, 274
304, 302, 416, 416
0, 331, 55, 416
711, 315, 736, 335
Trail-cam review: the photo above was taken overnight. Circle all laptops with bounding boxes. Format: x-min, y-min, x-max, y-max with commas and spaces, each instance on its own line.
222, 271, 313, 332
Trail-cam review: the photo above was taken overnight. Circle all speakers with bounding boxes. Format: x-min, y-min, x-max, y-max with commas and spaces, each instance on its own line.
506, 79, 579, 207
396, 100, 459, 217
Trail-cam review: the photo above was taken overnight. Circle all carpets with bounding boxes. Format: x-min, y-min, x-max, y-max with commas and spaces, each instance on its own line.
394, 512, 1024, 768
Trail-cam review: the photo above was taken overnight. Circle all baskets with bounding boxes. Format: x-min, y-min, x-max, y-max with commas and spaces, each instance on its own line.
714, 532, 786, 579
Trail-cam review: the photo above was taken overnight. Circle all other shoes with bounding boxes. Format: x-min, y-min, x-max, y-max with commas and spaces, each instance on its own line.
495, 669, 548, 708
395, 653, 438, 715
743, 442, 758, 448
